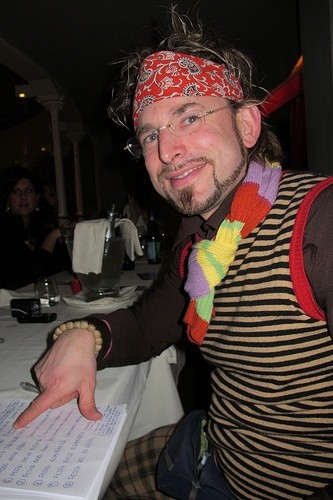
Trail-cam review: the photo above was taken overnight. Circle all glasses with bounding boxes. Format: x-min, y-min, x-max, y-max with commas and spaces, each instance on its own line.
124, 102, 238, 157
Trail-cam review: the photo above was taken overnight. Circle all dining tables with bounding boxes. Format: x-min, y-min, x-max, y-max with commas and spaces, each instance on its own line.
0, 245, 184, 500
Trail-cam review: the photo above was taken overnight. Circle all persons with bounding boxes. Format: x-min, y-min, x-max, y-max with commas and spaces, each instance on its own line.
13, 5, 333, 500
122, 194, 150, 237
0, 166, 73, 291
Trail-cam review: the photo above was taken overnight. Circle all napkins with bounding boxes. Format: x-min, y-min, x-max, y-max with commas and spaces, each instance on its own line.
0, 289, 60, 308
62, 285, 137, 311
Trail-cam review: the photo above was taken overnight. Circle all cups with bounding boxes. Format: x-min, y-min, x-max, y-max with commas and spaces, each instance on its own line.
34, 276, 60, 306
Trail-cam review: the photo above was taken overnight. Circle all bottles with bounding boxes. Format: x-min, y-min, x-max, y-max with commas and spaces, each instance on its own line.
147, 212, 161, 264
160, 232, 175, 269
106, 203, 116, 238
136, 214, 147, 248
115, 212, 135, 271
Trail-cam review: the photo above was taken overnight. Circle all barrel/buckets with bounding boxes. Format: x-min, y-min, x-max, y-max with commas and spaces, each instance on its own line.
63, 233, 126, 303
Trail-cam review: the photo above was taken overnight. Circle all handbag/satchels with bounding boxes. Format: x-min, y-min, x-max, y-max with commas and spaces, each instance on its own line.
154, 409, 239, 500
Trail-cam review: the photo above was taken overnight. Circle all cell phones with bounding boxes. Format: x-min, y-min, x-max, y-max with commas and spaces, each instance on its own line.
18, 312, 56, 323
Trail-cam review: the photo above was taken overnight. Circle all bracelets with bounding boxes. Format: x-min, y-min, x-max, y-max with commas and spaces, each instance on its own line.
59, 227, 66, 239
52, 320, 103, 358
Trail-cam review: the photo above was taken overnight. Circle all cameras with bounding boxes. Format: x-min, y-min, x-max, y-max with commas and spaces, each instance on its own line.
11, 298, 40, 318
